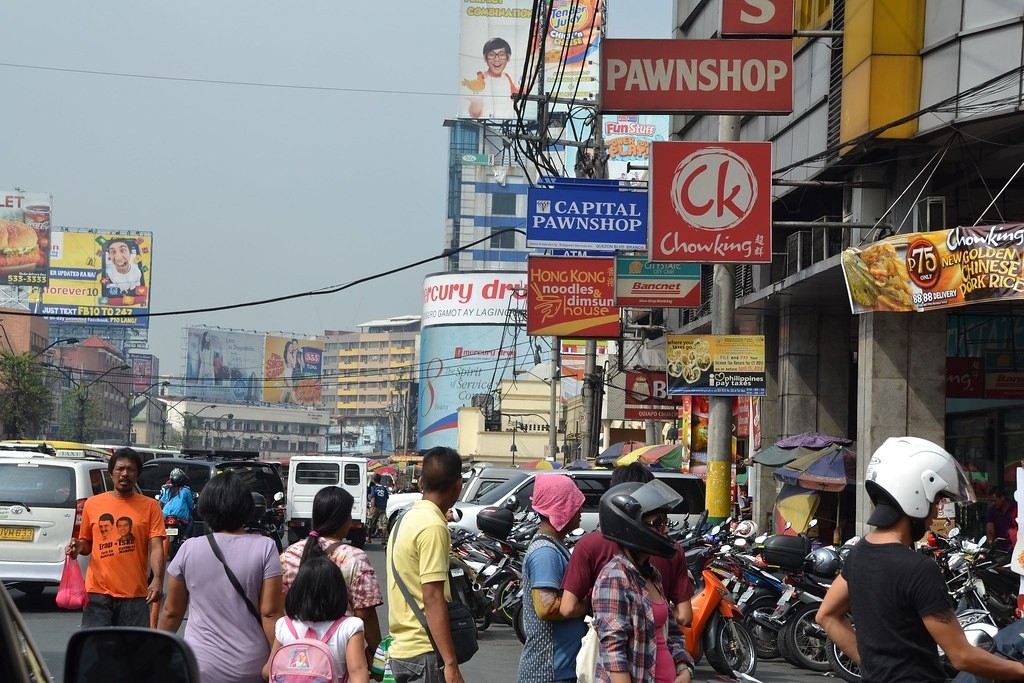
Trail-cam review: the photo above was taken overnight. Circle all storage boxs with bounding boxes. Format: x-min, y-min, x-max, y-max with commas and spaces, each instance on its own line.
759, 535, 807, 568
477, 507, 514, 541
921, 501, 956, 542
246, 492, 268, 523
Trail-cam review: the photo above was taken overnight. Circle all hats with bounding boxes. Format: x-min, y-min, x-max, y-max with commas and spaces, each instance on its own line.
866, 488, 901, 528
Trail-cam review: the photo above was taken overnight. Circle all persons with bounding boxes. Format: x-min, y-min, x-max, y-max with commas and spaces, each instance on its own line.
105, 237, 141, 290
516, 461, 695, 683
196, 330, 215, 386
280, 339, 301, 405
478, 37, 525, 119
65, 445, 471, 683
815, 436, 1024, 683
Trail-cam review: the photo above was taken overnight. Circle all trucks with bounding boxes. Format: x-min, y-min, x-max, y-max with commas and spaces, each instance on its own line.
285, 456, 367, 549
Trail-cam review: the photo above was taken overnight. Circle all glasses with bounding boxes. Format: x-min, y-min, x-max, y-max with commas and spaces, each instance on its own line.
643, 517, 672, 532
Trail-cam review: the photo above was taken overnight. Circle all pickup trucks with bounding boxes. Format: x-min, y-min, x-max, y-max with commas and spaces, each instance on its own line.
385, 470, 474, 536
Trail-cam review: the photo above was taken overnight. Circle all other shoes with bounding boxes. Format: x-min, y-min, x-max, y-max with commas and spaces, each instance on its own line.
180, 539, 187, 545
366, 537, 371, 543
381, 542, 387, 545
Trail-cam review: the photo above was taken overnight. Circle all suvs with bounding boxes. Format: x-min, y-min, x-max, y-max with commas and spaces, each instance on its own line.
136, 449, 284, 538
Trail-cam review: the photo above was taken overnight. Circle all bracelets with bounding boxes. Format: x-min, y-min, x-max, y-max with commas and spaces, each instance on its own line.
676, 665, 694, 679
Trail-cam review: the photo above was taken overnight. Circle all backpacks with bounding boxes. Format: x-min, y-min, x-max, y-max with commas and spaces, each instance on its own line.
575, 615, 601, 683
447, 554, 492, 630
269, 615, 349, 683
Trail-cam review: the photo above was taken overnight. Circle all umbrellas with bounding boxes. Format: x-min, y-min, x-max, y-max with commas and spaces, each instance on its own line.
520, 457, 562, 469
743, 432, 857, 543
565, 460, 593, 470
594, 440, 682, 470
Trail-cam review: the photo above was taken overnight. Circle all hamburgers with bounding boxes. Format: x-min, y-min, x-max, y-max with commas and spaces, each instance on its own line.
0, 219, 39, 276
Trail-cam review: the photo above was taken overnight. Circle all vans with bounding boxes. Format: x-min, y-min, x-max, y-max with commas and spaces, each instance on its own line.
455, 467, 535, 503
1, 440, 115, 461
0, 442, 144, 597
84, 442, 183, 465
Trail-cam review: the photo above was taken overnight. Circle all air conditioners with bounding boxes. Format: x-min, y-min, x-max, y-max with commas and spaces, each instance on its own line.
914, 196, 946, 234
786, 216, 842, 277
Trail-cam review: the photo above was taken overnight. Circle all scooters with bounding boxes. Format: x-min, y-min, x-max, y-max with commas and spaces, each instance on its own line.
243, 491, 288, 556
367, 503, 379, 537
154, 490, 200, 562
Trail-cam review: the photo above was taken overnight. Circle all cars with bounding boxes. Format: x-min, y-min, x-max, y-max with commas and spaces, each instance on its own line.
447, 467, 707, 542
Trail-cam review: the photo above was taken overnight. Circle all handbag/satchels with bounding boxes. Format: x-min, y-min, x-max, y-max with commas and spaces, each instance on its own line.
424, 599, 479, 668
56, 553, 89, 609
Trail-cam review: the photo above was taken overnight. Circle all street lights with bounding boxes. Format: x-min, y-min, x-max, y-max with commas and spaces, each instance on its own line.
95, 380, 171, 446
39, 361, 132, 442
515, 369, 556, 463
220, 428, 282, 450
182, 409, 234, 449
135, 393, 197, 451
0, 337, 81, 439
160, 404, 217, 448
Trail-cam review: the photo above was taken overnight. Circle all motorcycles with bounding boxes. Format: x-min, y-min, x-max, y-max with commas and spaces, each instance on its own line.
449, 496, 1023, 683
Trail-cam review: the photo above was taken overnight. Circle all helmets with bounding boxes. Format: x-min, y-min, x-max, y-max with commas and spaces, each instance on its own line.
251, 491, 266, 507
864, 437, 968, 520
170, 468, 189, 483
599, 482, 677, 559
734, 520, 759, 539
805, 547, 841, 576
